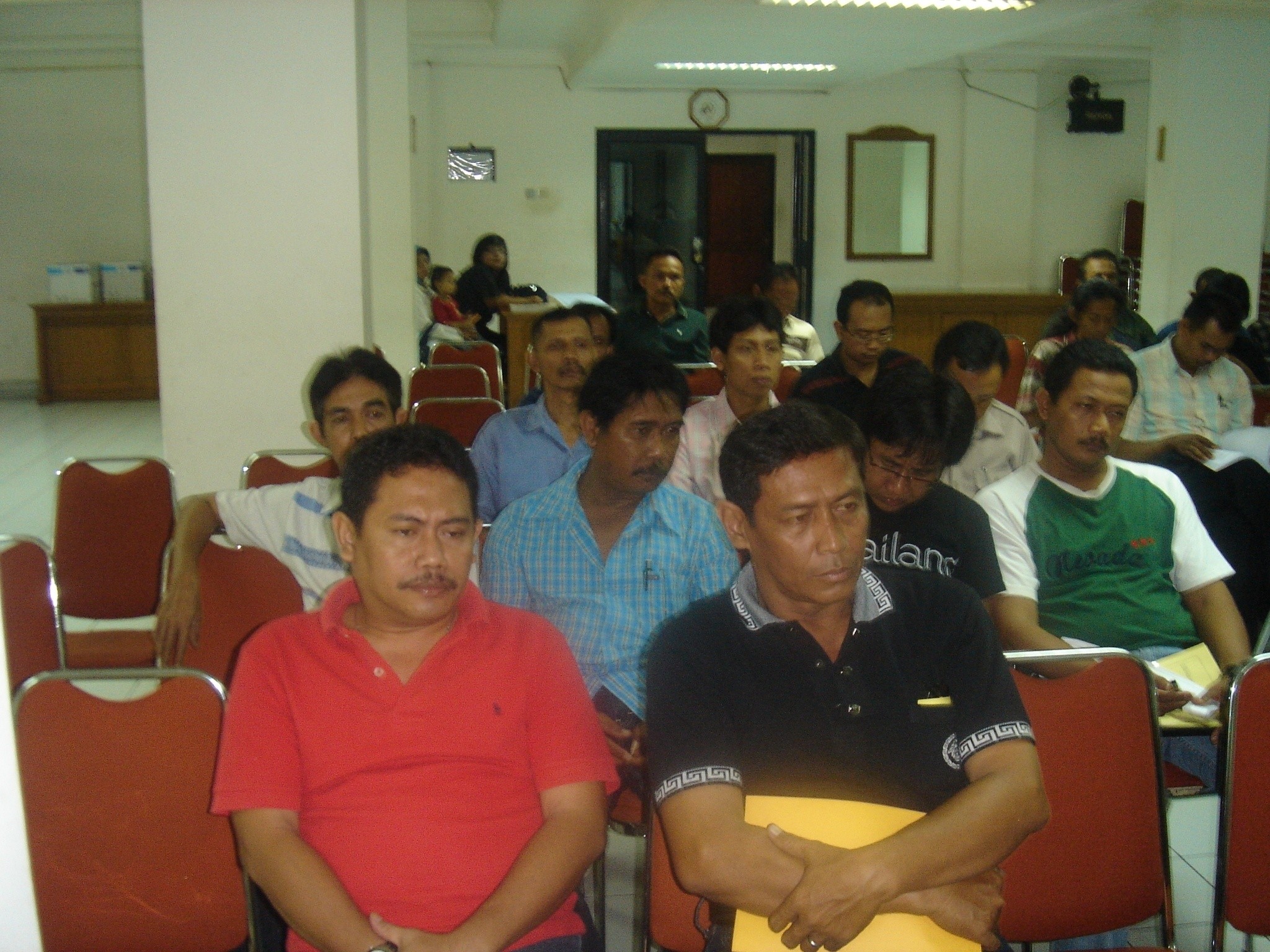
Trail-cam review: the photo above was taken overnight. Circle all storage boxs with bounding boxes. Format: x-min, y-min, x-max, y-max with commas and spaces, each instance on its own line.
45, 261, 147, 306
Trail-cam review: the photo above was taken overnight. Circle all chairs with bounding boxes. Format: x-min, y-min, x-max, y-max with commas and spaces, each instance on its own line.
49, 451, 180, 670
1209, 651, 1270, 951
637, 760, 712, 950
1057, 198, 1144, 312
239, 446, 341, 488
995, 333, 1029, 409
407, 343, 817, 458
155, 523, 303, 686
12, 669, 266, 952
996, 644, 1178, 951
0, 534, 69, 691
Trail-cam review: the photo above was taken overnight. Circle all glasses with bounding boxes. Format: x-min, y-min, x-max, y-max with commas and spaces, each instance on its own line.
841, 324, 894, 344
867, 439, 944, 489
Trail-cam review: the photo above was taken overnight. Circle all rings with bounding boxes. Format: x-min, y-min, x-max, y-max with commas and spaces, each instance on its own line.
807, 936, 819, 949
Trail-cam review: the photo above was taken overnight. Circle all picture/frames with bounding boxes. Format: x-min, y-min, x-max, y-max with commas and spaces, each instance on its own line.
689, 87, 732, 130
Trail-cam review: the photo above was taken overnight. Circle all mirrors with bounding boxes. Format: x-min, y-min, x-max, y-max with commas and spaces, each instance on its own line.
846, 126, 936, 263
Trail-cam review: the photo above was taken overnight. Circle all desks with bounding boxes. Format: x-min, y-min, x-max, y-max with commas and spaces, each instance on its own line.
30, 302, 162, 403
496, 295, 564, 413
886, 293, 1063, 365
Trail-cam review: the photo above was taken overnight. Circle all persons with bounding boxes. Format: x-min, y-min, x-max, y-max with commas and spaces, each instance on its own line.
635, 393, 1053, 952
660, 295, 786, 564
475, 346, 743, 809
151, 346, 402, 670
749, 260, 825, 362
417, 232, 545, 364
847, 354, 1006, 604
469, 302, 618, 523
970, 335, 1251, 793
209, 418, 623, 952
1017, 246, 1270, 654
612, 248, 712, 362
929, 324, 1038, 500
794, 281, 928, 420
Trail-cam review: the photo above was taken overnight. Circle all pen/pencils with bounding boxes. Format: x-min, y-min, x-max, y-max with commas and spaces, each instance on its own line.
1013, 663, 1047, 680
1171, 679, 1182, 710
